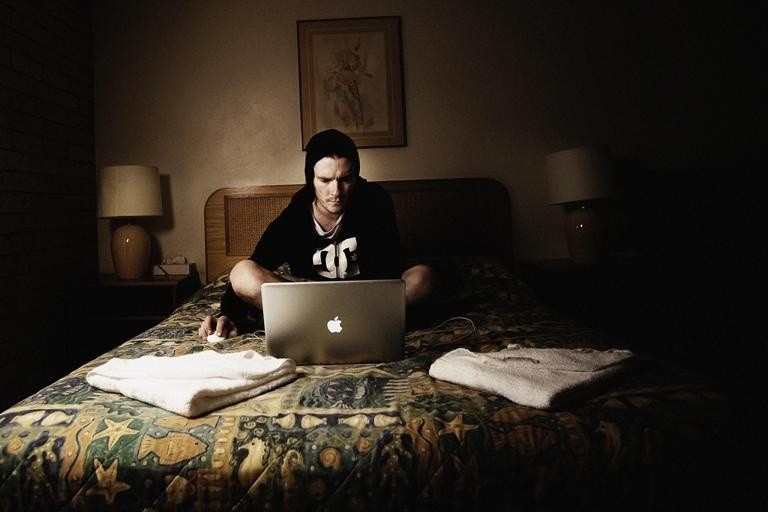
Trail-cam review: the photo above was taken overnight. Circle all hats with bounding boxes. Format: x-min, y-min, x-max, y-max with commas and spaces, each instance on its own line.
305, 128, 361, 189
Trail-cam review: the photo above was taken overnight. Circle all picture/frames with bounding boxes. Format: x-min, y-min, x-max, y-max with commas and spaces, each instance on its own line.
296, 15, 407, 151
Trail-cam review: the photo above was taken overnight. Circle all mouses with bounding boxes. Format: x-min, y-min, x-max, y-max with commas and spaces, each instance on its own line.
206, 329, 227, 344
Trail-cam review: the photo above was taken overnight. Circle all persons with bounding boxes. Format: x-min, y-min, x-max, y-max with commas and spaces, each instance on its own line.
197, 128, 440, 340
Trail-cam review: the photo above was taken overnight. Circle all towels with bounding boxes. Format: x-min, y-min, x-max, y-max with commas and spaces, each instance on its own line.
428, 345, 634, 412
87, 348, 297, 418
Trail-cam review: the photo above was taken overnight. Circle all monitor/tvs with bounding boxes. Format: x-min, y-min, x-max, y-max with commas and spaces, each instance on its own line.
259, 278, 408, 366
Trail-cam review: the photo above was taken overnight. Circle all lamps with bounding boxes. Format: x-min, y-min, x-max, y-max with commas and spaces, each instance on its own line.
94, 165, 163, 280
546, 146, 609, 265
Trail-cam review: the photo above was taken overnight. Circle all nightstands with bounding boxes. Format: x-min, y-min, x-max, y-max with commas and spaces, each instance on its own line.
102, 274, 199, 346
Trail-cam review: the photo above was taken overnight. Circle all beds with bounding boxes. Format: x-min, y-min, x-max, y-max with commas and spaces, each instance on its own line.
0, 180, 712, 512
